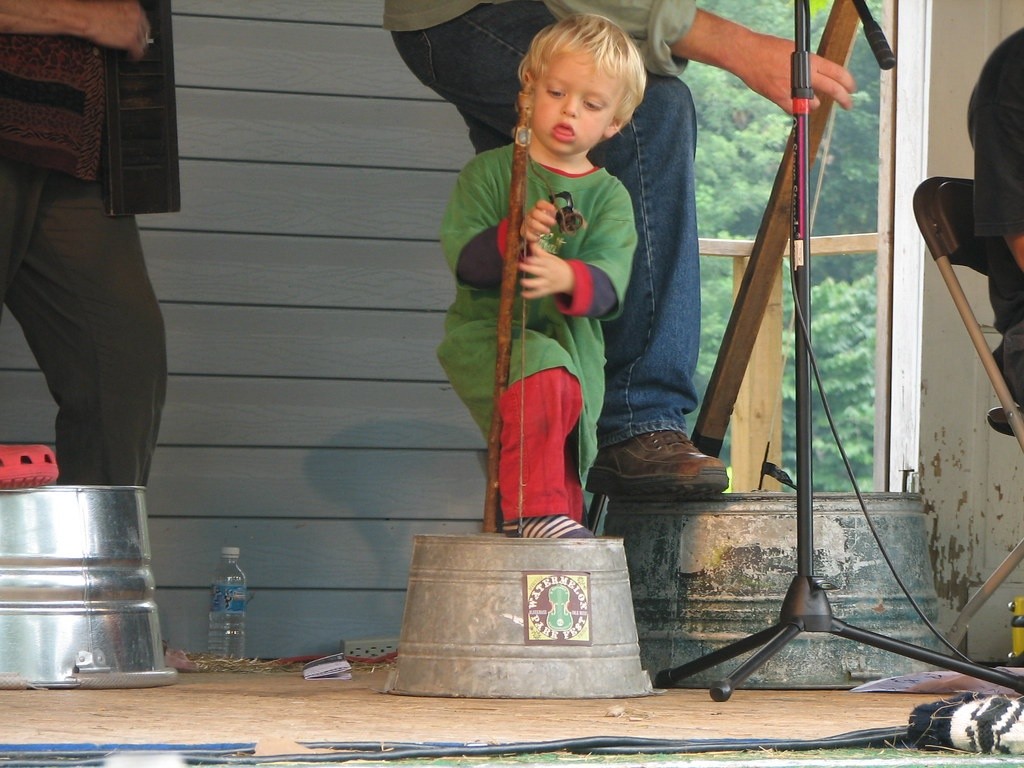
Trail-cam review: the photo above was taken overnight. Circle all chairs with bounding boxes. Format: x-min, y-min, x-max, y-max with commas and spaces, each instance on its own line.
911, 175, 1022, 655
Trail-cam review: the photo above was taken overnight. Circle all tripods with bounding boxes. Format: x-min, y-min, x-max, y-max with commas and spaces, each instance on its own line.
653, 0, 1024, 703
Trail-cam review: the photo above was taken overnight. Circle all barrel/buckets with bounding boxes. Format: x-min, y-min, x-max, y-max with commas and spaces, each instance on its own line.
0, 485, 179, 689
386, 532, 663, 699
600, 489, 950, 692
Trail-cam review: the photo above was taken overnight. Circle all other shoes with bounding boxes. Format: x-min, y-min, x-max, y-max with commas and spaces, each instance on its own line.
585, 429, 729, 496
986, 407, 1016, 437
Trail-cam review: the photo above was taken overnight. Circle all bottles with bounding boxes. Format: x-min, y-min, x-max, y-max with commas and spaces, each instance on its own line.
207, 547, 247, 659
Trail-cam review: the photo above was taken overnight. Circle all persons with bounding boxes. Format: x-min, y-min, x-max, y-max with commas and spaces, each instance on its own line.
435, 13, 647, 539
0, 0, 180, 487
383, 0, 853, 498
969, 28, 1024, 407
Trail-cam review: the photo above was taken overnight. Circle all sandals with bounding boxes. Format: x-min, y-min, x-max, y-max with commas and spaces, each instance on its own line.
0, 444, 60, 489
